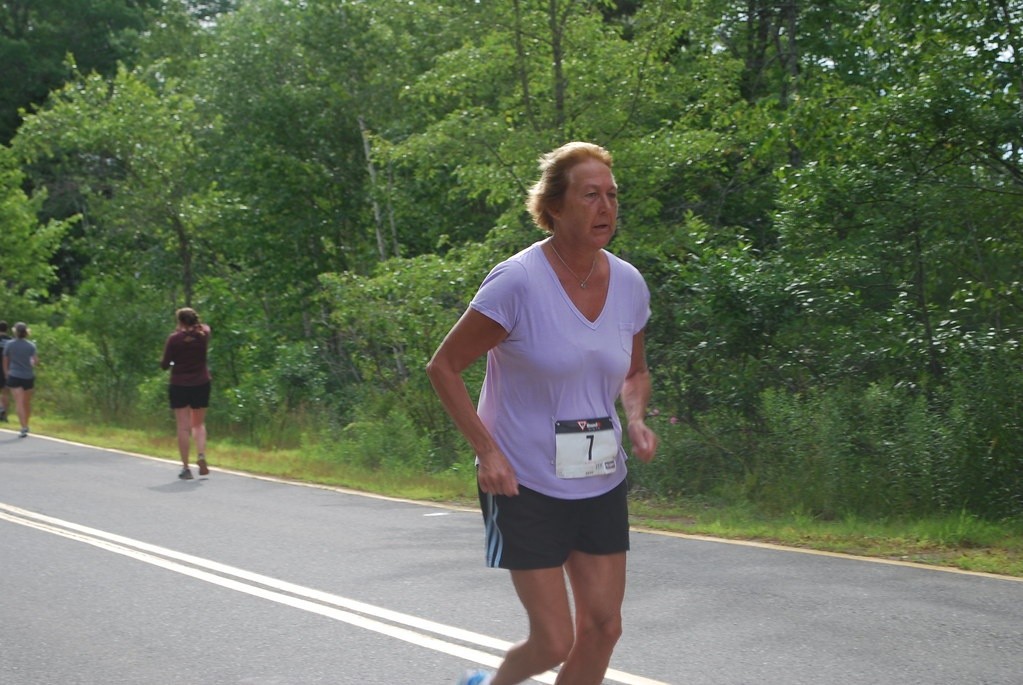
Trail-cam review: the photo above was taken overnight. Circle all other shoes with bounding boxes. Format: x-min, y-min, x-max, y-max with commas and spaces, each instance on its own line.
18, 427, 28, 438
460, 671, 494, 685
178, 469, 193, 479
0, 407, 5, 420
196, 456, 209, 475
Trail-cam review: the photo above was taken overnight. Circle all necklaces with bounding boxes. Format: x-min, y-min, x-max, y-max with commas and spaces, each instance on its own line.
549, 238, 596, 289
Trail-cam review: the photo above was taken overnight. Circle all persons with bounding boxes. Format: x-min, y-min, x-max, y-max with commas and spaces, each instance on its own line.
0, 320, 39, 437
426, 142, 657, 685
160, 307, 212, 479
